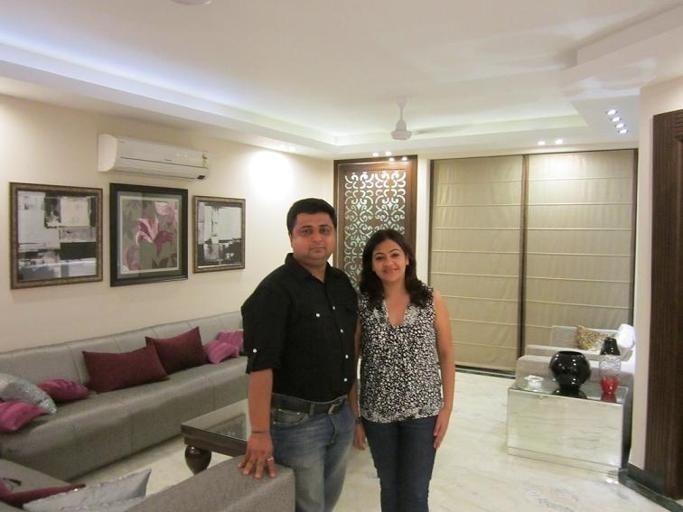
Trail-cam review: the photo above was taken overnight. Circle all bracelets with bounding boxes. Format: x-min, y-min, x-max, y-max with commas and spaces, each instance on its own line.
356, 417, 365, 426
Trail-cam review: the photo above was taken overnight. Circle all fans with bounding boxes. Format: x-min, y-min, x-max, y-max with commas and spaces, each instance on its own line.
333, 95, 473, 142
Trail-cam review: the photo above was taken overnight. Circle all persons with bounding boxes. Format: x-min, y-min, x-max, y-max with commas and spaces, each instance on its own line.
347, 228, 455, 512
237, 197, 359, 512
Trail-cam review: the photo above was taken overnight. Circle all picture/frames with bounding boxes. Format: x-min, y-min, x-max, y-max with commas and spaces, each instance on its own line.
109, 182, 188, 288
191, 195, 246, 273
9, 182, 103, 289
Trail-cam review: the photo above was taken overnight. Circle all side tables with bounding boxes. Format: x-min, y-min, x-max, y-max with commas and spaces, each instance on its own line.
506, 375, 629, 477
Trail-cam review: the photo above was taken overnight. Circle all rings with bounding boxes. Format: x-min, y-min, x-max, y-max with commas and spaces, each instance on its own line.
265, 457, 274, 463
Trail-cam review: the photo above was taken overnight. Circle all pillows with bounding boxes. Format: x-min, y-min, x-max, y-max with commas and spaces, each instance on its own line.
575, 324, 599, 349
203, 340, 239, 364
218, 331, 244, 354
144, 326, 209, 373
23, 469, 151, 511
82, 342, 170, 395
1, 401, 51, 433
1, 480, 85, 505
35, 378, 97, 402
1, 373, 58, 415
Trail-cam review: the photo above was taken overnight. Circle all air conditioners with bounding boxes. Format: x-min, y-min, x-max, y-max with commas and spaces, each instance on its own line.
96, 132, 210, 182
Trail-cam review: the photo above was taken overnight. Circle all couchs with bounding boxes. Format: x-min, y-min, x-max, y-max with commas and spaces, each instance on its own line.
0, 455, 295, 512
514, 323, 635, 448
1, 311, 251, 483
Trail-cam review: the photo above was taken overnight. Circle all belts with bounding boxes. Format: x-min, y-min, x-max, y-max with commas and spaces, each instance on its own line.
272, 396, 347, 416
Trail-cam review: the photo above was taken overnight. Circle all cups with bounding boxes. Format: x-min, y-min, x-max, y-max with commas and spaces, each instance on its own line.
524, 375, 544, 393
600, 358, 621, 404
